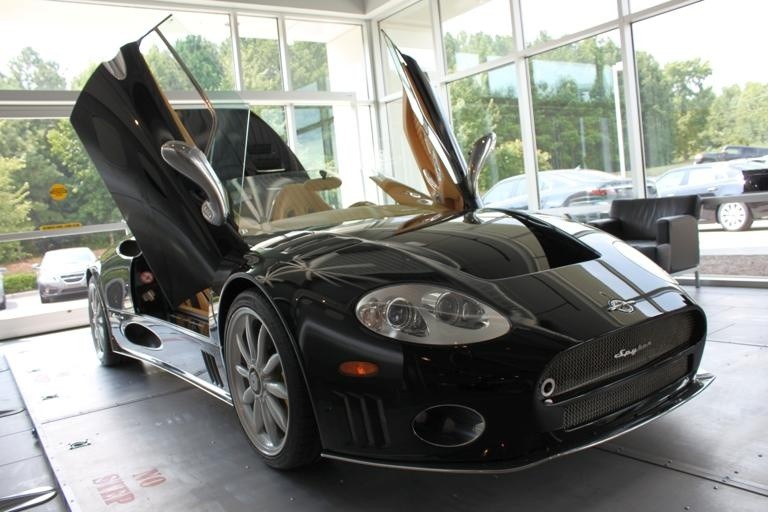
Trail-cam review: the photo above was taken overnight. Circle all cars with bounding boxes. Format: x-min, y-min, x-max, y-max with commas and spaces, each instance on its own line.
480, 145, 768, 234
0, 247, 100, 309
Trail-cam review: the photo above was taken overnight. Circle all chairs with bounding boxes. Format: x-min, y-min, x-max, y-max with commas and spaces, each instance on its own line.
584, 196, 700, 294
271, 183, 331, 222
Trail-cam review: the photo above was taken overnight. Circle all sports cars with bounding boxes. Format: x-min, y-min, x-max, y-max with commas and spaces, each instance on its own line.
69, 11, 716, 474
